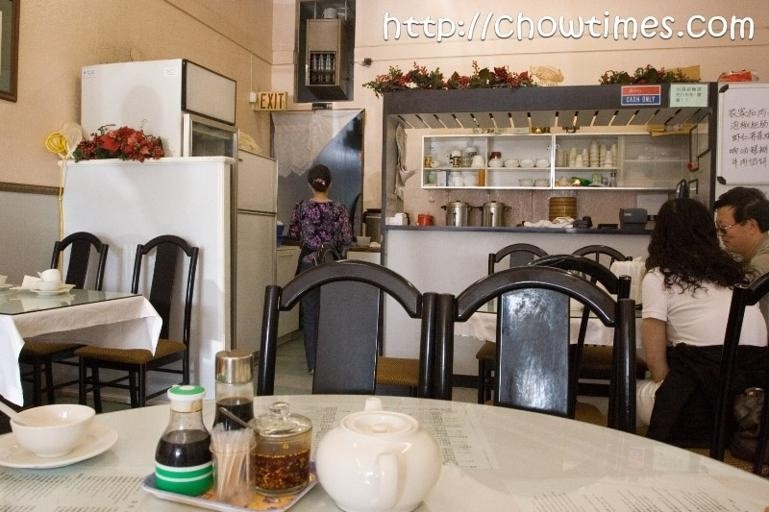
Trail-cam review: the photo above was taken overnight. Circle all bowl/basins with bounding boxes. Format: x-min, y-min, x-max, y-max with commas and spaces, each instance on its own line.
0, 275, 7, 285
7, 404, 96, 460
505, 159, 550, 168
518, 179, 548, 186
40, 281, 60, 290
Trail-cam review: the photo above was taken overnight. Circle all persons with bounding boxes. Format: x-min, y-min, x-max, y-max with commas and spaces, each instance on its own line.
713, 187, 769, 337
288, 165, 352, 374
635, 199, 769, 450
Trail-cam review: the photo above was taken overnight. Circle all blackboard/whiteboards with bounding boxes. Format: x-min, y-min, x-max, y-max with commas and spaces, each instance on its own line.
716, 81, 769, 186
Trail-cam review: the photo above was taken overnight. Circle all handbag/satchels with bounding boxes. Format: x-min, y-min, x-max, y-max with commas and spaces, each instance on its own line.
727, 384, 768, 466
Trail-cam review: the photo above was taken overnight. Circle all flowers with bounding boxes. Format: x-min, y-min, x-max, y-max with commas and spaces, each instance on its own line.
72, 123, 164, 163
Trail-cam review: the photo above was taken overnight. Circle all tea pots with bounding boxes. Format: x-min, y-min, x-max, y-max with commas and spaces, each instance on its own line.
315, 396, 441, 512
37, 269, 61, 282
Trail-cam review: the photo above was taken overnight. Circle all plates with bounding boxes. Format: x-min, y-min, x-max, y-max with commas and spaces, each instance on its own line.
141, 460, 320, 511
0, 428, 117, 470
0, 284, 13, 289
548, 196, 578, 222
30, 286, 70, 297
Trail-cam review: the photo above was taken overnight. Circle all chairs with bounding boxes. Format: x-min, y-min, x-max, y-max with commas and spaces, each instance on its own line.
75, 235, 199, 405
18, 231, 109, 406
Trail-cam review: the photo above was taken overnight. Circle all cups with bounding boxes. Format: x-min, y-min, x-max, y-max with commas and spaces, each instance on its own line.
418, 214, 433, 226
557, 139, 617, 168
446, 172, 479, 187
207, 439, 259, 507
424, 155, 432, 168
322, 7, 337, 19
356, 236, 371, 249
473, 155, 485, 168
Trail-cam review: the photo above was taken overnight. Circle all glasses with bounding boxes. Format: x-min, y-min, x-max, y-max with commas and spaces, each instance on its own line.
716, 221, 738, 235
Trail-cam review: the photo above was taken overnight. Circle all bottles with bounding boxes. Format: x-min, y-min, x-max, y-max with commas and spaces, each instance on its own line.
244, 401, 314, 494
152, 383, 214, 497
311, 54, 336, 86
479, 169, 484, 186
489, 156, 503, 167
610, 172, 617, 187
212, 349, 257, 433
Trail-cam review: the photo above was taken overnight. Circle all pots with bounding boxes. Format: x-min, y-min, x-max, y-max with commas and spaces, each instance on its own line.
364, 207, 384, 244
440, 200, 511, 227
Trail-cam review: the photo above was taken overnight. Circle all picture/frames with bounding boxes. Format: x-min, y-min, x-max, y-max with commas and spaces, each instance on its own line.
688, 112, 712, 171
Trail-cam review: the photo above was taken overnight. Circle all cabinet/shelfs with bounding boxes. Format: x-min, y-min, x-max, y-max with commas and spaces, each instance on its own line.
420, 134, 692, 190
304, 19, 348, 99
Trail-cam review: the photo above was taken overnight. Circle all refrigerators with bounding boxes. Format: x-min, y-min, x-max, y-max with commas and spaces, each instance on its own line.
78, 58, 238, 348
